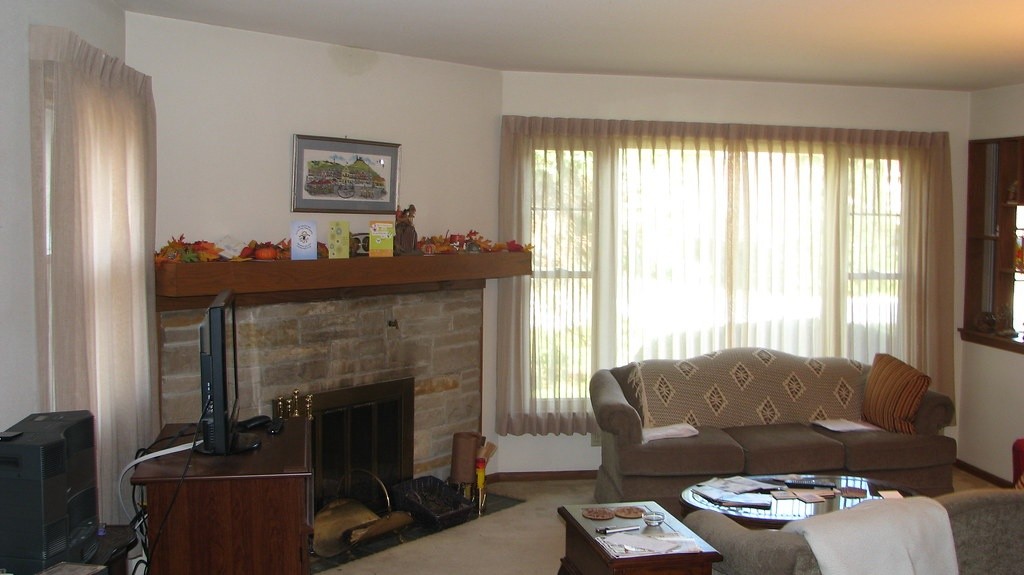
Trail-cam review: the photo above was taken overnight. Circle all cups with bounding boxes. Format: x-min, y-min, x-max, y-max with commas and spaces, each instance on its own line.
996, 306, 1012, 335
449, 234, 465, 250
1006, 185, 1019, 204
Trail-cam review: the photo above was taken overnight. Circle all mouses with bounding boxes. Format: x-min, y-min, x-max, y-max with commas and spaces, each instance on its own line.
241, 416, 273, 431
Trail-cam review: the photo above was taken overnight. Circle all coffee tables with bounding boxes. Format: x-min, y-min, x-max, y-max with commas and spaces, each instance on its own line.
557, 501, 723, 575
669, 475, 918, 529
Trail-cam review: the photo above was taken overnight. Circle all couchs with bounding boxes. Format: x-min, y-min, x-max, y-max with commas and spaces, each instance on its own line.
683, 439, 1024, 575
589, 347, 958, 497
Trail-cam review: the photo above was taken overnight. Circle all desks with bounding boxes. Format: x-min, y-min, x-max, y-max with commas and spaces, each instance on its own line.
130, 415, 312, 575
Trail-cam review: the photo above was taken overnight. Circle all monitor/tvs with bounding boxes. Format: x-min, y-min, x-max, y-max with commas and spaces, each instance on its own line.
199, 288, 240, 453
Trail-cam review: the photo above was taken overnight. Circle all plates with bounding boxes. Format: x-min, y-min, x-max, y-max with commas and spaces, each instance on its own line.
583, 507, 614, 520
615, 506, 646, 518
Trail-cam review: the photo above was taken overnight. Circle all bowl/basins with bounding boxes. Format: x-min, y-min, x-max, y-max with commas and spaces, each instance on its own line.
642, 512, 665, 526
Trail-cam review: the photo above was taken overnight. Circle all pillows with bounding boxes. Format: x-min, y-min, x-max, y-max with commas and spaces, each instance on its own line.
860, 353, 931, 434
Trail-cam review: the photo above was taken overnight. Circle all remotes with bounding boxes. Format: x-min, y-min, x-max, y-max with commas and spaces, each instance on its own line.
268, 418, 285, 434
785, 480, 836, 490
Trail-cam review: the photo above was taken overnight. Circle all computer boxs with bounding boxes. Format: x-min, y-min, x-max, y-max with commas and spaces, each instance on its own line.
0, 409, 99, 575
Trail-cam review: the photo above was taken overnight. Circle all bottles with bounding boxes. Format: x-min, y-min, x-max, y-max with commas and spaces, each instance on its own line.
476, 458, 486, 489
1015, 236, 1024, 272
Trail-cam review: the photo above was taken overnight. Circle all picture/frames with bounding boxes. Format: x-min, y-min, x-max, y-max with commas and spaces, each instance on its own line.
291, 135, 402, 215
